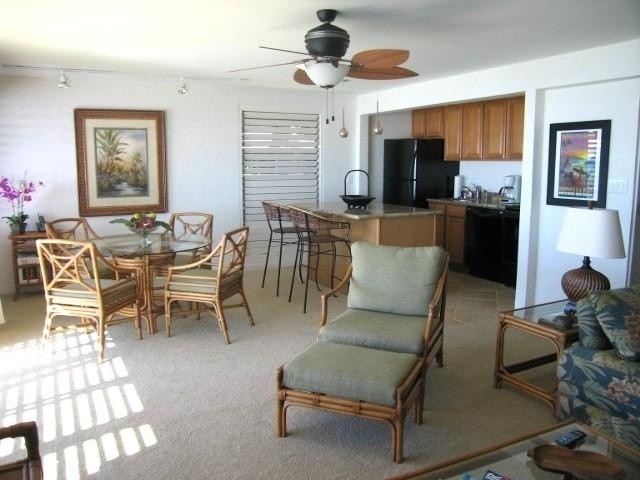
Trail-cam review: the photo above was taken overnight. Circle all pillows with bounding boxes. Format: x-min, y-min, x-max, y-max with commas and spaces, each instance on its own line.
347, 241, 446, 319
588, 286, 640, 361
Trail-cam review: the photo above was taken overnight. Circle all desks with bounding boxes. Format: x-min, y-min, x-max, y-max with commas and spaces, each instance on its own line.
8, 229, 62, 302
293, 202, 443, 296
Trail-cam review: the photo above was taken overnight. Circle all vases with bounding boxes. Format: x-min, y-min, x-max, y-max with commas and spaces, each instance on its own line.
9, 222, 27, 236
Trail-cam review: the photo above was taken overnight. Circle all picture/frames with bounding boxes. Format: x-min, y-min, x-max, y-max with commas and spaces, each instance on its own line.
546, 120, 612, 208
73, 108, 169, 217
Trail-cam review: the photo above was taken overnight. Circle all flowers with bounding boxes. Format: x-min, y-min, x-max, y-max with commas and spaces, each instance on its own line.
0, 175, 38, 223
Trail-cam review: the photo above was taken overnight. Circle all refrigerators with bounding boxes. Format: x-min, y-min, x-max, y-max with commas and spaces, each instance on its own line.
383, 138, 460, 208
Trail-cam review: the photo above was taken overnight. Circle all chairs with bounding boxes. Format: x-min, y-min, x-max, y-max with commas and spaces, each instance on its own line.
35, 211, 255, 365
316, 252, 450, 421
287, 204, 352, 314
0, 422, 44, 480
261, 199, 323, 296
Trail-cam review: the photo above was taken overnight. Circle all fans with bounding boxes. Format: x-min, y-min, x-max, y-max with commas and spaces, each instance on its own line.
229, 9, 419, 86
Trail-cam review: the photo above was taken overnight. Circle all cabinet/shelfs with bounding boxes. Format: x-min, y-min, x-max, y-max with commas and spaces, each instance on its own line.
482, 96, 525, 160
443, 102, 483, 162
429, 202, 466, 265
411, 107, 443, 138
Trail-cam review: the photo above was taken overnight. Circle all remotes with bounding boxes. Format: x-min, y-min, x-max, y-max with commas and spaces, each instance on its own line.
555, 429, 587, 447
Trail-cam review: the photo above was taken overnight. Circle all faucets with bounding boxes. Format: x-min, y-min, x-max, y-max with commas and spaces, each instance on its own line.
461, 183, 481, 198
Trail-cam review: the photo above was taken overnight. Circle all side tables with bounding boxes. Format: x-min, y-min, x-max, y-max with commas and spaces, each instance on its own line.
494, 298, 579, 418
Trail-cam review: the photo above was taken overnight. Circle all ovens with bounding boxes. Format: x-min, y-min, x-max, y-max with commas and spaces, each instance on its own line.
501, 209, 520, 288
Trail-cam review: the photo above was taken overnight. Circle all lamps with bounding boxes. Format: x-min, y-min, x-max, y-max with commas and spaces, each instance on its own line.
339, 107, 348, 138
373, 100, 384, 135
177, 80, 188, 96
556, 207, 626, 302
304, 61, 350, 124
58, 72, 70, 90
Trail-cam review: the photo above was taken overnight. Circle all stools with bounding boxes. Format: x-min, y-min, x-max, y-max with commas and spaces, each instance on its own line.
275, 341, 425, 463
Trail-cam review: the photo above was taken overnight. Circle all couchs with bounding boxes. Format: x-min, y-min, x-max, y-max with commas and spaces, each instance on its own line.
557, 297, 640, 456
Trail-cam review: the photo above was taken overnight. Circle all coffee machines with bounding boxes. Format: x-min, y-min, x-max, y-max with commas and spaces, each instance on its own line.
498, 175, 520, 204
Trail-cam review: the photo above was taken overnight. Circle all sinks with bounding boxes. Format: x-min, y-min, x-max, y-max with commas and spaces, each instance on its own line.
453, 198, 487, 203
